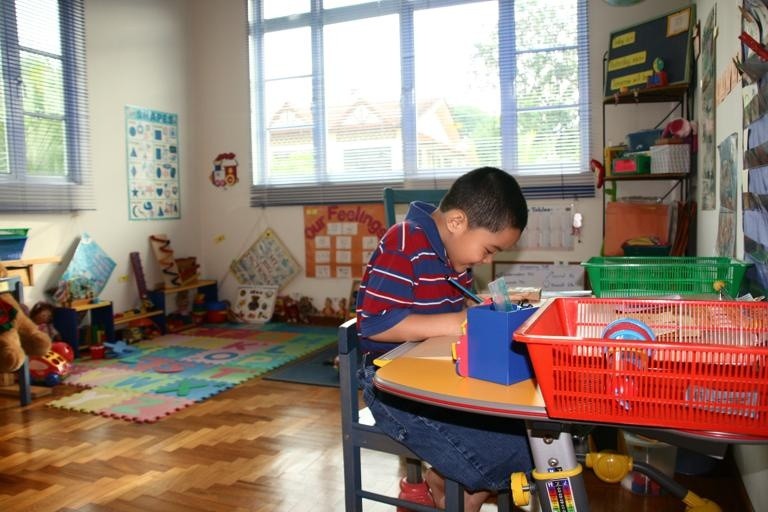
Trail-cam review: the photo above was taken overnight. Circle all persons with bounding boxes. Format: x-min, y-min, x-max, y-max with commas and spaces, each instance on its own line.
353, 165, 540, 511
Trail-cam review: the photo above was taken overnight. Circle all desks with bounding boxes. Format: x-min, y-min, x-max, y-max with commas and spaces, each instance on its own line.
0, 256, 62, 399
374, 294, 767, 512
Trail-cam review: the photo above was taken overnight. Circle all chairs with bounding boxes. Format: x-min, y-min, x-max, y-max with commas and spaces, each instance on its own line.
383, 187, 449, 233
338, 317, 511, 512
0, 275, 32, 406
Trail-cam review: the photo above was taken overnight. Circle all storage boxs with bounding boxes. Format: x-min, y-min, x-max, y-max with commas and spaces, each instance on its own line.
0, 238, 29, 260
0, 228, 30, 237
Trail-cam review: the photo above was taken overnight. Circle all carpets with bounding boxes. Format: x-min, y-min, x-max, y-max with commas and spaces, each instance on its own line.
262, 344, 339, 387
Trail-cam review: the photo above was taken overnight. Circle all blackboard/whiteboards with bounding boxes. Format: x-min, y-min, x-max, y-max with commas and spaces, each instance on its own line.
603, 2, 697, 99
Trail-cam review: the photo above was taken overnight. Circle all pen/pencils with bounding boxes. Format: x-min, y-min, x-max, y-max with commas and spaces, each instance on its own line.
447, 276, 484, 304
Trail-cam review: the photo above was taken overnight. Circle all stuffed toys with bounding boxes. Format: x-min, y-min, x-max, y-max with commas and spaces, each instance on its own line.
0, 262, 44, 370
646, 56, 672, 90
660, 117, 697, 141
153, 291, 353, 333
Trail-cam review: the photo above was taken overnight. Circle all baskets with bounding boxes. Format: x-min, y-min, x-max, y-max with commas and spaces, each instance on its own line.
512, 296, 768, 437
580, 255, 756, 301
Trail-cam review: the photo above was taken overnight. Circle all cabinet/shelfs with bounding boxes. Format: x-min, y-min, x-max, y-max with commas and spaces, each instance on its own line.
53, 279, 218, 359
603, 50, 695, 259
